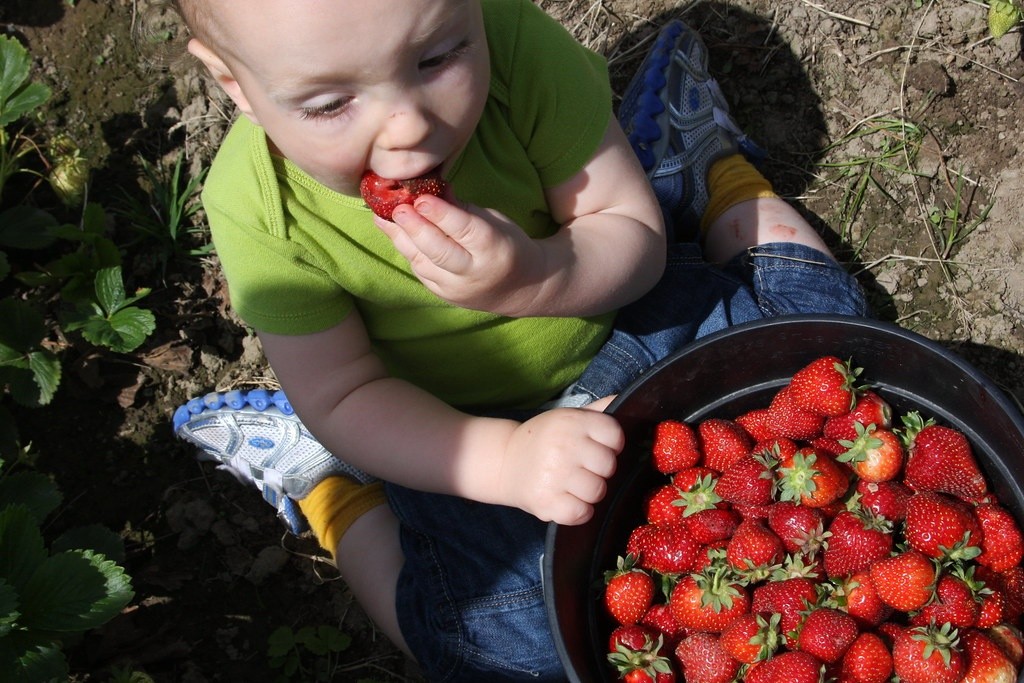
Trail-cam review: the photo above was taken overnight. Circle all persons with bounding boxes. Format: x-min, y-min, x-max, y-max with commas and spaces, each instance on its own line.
166, 0, 872, 683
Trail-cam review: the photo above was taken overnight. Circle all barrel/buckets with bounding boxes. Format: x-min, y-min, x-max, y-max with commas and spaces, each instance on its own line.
544, 312, 1024, 683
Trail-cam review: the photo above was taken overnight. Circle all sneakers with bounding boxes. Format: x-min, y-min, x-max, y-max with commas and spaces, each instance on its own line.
616, 18, 757, 241
173, 389, 378, 536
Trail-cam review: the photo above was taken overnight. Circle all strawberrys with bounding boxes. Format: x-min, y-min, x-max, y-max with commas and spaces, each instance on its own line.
360, 170, 442, 223
51, 160, 91, 209
602, 355, 1024, 682
